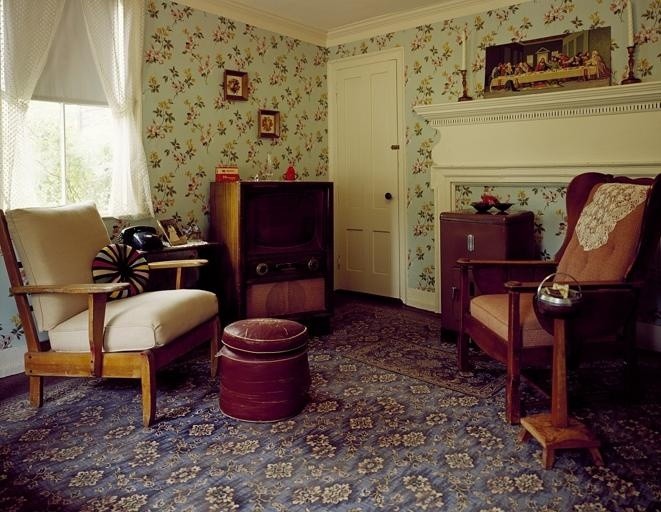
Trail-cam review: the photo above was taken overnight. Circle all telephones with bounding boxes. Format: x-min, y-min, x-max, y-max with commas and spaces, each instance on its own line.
120, 225, 163, 251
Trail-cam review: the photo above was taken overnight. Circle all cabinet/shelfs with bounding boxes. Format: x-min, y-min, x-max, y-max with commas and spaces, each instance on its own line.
207, 180, 335, 322
439, 211, 536, 341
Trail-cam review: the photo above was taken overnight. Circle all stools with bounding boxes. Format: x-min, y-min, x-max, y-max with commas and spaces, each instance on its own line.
219, 319, 311, 423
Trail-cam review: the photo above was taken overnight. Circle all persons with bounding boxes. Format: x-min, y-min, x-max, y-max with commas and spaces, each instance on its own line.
491, 51, 610, 90
167, 223, 182, 241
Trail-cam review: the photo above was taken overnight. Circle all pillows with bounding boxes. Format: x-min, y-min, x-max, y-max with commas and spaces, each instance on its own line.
90, 241, 148, 300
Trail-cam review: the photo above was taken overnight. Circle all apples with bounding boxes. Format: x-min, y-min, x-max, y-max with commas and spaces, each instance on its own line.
489, 199, 498, 204
483, 195, 494, 204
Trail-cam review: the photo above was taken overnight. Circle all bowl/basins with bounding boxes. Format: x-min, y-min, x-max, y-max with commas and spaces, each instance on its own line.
470, 201, 514, 213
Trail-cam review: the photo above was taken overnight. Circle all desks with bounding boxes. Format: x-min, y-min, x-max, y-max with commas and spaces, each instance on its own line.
119, 239, 209, 289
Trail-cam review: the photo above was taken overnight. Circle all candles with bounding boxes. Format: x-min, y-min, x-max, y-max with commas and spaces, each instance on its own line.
461, 28, 467, 69
627, 0, 634, 46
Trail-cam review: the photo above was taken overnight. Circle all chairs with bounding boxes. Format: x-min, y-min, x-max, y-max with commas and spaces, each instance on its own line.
458, 172, 660, 424
0, 202, 222, 428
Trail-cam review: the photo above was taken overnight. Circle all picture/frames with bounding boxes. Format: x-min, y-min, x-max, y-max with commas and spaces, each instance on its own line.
156, 217, 184, 245
222, 69, 248, 101
257, 110, 280, 139
